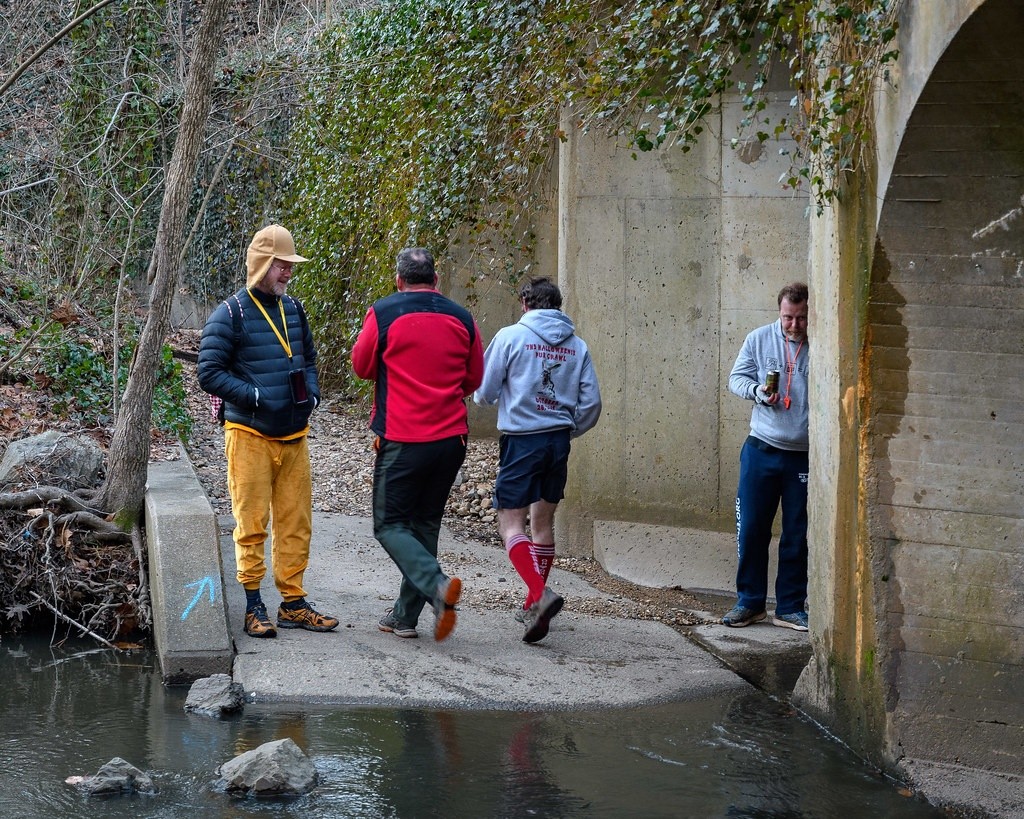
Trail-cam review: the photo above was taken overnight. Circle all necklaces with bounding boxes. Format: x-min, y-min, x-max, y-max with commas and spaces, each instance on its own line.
783, 337, 803, 409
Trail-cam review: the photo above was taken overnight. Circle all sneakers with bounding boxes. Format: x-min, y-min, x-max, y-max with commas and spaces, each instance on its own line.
723, 605, 767, 628
522, 588, 564, 643
772, 608, 810, 630
377, 606, 418, 638
244, 605, 278, 638
434, 578, 461, 640
515, 604, 530, 624
276, 603, 339, 631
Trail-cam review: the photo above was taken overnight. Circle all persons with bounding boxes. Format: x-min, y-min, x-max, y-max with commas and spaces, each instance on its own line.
470, 276, 602, 643
196, 223, 341, 636
348, 247, 484, 641
720, 282, 809, 632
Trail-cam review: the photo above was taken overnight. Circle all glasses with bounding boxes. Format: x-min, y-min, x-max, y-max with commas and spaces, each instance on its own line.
271, 263, 295, 275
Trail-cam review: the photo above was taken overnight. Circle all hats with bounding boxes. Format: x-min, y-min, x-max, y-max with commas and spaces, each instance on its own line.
246, 224, 311, 291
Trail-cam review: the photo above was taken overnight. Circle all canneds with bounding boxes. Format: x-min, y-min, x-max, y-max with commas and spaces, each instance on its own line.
765, 370, 779, 397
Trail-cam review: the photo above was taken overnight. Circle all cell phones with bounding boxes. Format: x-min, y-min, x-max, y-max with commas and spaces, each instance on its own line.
289, 369, 308, 404
